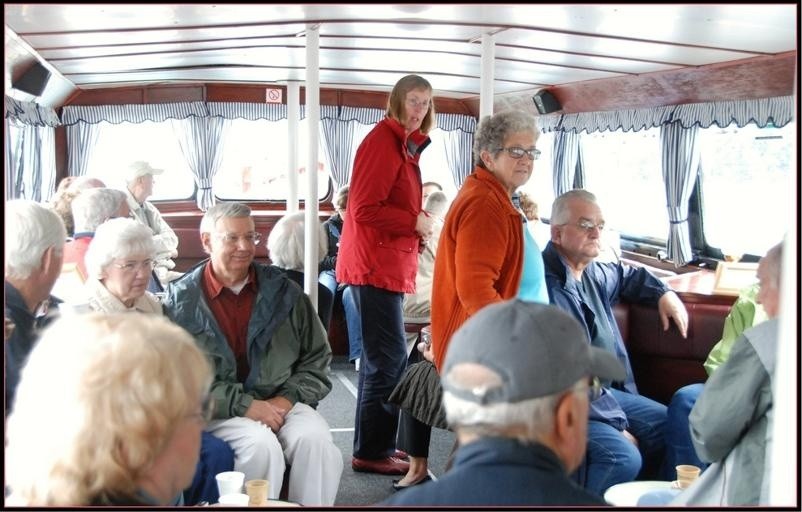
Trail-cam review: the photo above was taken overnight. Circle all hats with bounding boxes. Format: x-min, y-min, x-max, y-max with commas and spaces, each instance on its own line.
442, 298, 627, 404
125, 162, 164, 180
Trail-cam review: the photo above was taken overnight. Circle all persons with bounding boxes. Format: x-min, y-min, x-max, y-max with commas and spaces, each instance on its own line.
428, 110, 546, 476
662, 281, 768, 481
679, 236, 784, 512
333, 74, 434, 476
517, 191, 552, 254
370, 298, 626, 507
541, 189, 689, 498
322, 184, 362, 373
2, 163, 345, 509
400, 192, 447, 365
421, 181, 444, 212
387, 339, 445, 491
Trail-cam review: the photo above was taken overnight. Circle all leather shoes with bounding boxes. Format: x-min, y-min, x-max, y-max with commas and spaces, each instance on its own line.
393, 475, 432, 490
351, 450, 410, 474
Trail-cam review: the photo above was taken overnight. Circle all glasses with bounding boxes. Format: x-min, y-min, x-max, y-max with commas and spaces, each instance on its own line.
113, 257, 153, 273
494, 145, 541, 160
219, 232, 262, 242
407, 97, 430, 109
560, 218, 605, 230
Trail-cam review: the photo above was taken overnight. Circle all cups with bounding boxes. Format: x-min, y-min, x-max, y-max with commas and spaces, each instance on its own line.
215, 471, 269, 508
676, 465, 701, 489
